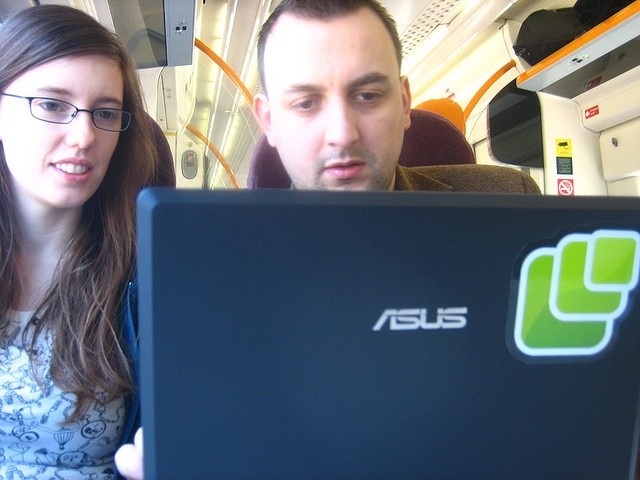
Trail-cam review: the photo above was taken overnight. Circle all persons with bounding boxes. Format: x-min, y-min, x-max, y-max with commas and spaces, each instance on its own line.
0, 6, 158, 479
114, 0, 542, 480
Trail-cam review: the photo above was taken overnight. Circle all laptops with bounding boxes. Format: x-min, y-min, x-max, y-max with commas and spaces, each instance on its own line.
135, 187, 640, 480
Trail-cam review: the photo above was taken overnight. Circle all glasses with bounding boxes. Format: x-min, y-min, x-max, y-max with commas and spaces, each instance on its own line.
0, 94, 133, 132
